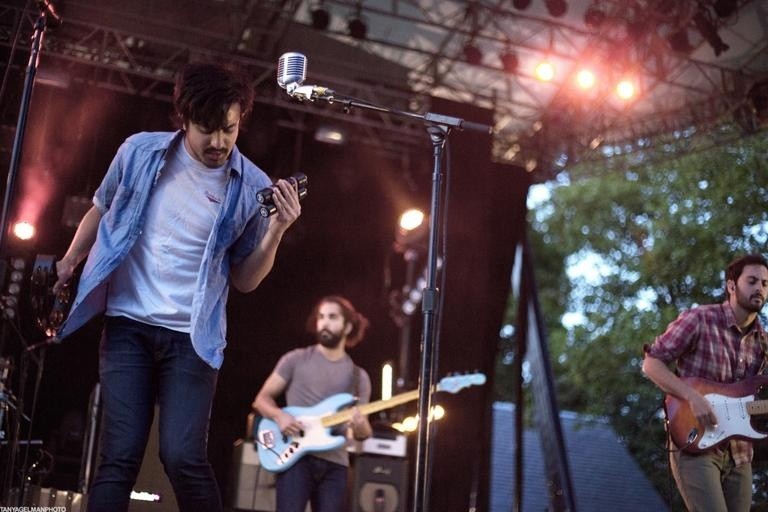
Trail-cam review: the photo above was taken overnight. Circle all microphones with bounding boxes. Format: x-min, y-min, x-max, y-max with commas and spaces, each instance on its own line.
276, 51, 331, 102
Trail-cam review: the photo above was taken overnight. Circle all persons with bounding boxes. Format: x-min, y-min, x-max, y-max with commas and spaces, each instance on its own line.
56, 52, 300, 510
245, 289, 373, 512
634, 251, 767, 511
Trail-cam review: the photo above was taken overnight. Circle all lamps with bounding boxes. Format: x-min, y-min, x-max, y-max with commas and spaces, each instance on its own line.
310, 0, 739, 103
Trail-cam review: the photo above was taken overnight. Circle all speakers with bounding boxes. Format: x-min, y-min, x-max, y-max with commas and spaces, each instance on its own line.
227, 441, 312, 512
350, 452, 415, 512
79, 382, 179, 512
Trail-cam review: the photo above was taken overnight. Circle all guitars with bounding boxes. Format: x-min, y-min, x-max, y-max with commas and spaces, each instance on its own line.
257, 369, 487, 472
665, 374, 768, 456
